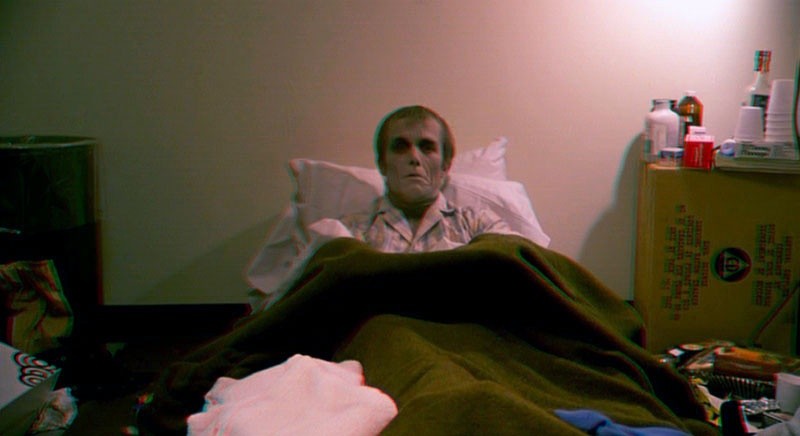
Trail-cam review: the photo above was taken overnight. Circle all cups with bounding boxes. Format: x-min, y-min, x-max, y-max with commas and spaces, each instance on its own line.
765, 79, 794, 142
733, 106, 764, 143
684, 142, 714, 169
777, 372, 800, 414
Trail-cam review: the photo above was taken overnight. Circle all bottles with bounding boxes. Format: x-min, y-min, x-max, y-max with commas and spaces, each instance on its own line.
645, 91, 703, 158
743, 49, 772, 107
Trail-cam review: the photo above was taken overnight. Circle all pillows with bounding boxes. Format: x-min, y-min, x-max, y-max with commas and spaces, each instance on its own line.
284, 136, 552, 261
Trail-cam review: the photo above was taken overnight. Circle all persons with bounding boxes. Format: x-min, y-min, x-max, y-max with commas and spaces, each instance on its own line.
311, 103, 515, 252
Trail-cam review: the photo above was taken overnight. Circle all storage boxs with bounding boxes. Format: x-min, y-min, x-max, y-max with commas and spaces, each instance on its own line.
633, 156, 800, 362
